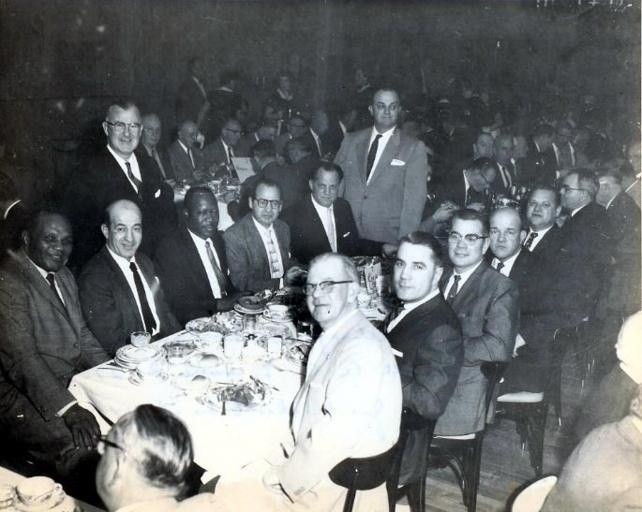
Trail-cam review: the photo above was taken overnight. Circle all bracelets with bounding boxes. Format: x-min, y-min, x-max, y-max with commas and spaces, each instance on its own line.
129, 330, 187, 386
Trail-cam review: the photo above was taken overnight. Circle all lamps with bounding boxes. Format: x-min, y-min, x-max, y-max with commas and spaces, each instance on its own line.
493, 323, 575, 478
394, 411, 438, 512
427, 360, 509, 512
330, 406, 407, 512
507, 471, 557, 512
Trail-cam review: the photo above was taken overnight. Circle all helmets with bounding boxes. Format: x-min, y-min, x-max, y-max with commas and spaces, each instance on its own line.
115, 303, 295, 414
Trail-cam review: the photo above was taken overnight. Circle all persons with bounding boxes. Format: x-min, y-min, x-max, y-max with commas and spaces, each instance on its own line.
96, 403, 215, 511
552, 308, 641, 457
62, 99, 180, 258
2, 210, 108, 475
520, 187, 597, 356
168, 120, 205, 186
201, 118, 241, 182
422, 157, 498, 223
79, 201, 183, 358
255, 254, 405, 510
278, 163, 396, 265
487, 208, 563, 394
424, 208, 522, 440
151, 186, 254, 330
224, 180, 307, 294
333, 85, 428, 245
134, 109, 171, 193
540, 388, 637, 510
184, 62, 642, 309
355, 232, 464, 482
560, 167, 617, 307
225, 140, 311, 222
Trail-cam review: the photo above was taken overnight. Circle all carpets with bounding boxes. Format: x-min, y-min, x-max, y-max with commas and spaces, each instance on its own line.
366, 134, 383, 181
265, 231, 282, 279
46, 273, 66, 312
496, 263, 505, 273
524, 232, 538, 250
327, 209, 335, 250
186, 149, 194, 168
125, 163, 142, 189
445, 275, 462, 303
206, 241, 227, 293
130, 262, 156, 334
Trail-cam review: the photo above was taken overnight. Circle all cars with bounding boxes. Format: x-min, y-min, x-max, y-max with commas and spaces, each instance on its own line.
135, 305, 289, 376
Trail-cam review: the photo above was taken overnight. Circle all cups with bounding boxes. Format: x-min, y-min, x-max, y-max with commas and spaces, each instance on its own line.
481, 173, 493, 186
562, 186, 583, 193
502, 167, 510, 191
256, 198, 281, 209
107, 120, 142, 133
302, 279, 354, 294
447, 231, 488, 245
223, 127, 244, 137
